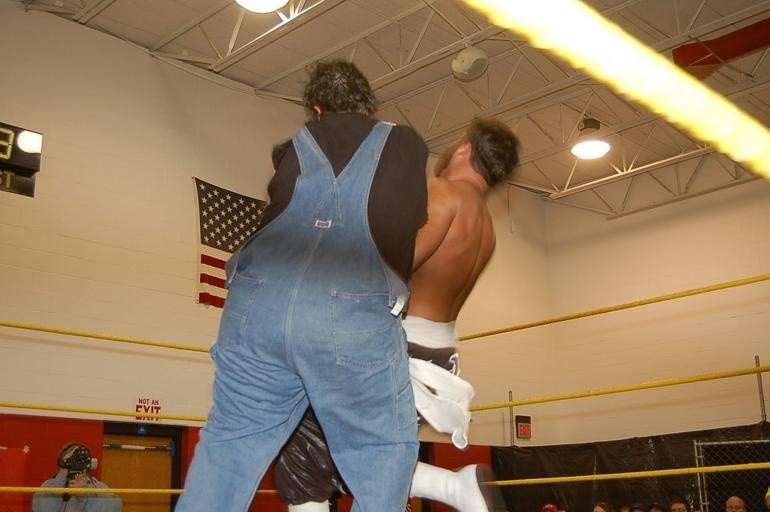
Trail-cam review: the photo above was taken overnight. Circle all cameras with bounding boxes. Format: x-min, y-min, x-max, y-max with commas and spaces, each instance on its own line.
62, 447, 98, 480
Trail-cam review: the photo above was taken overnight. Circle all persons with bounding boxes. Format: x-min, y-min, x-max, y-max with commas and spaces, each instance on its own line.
167, 56, 429, 512
269, 113, 525, 512
540, 485, 770, 511
30, 439, 124, 511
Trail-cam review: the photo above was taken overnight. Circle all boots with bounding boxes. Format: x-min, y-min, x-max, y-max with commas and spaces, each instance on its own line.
404, 460, 511, 512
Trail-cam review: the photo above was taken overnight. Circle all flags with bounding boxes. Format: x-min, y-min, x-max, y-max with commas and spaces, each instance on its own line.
195, 175, 270, 310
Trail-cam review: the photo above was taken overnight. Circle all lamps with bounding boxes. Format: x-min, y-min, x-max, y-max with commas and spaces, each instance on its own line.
571, 116, 612, 161
